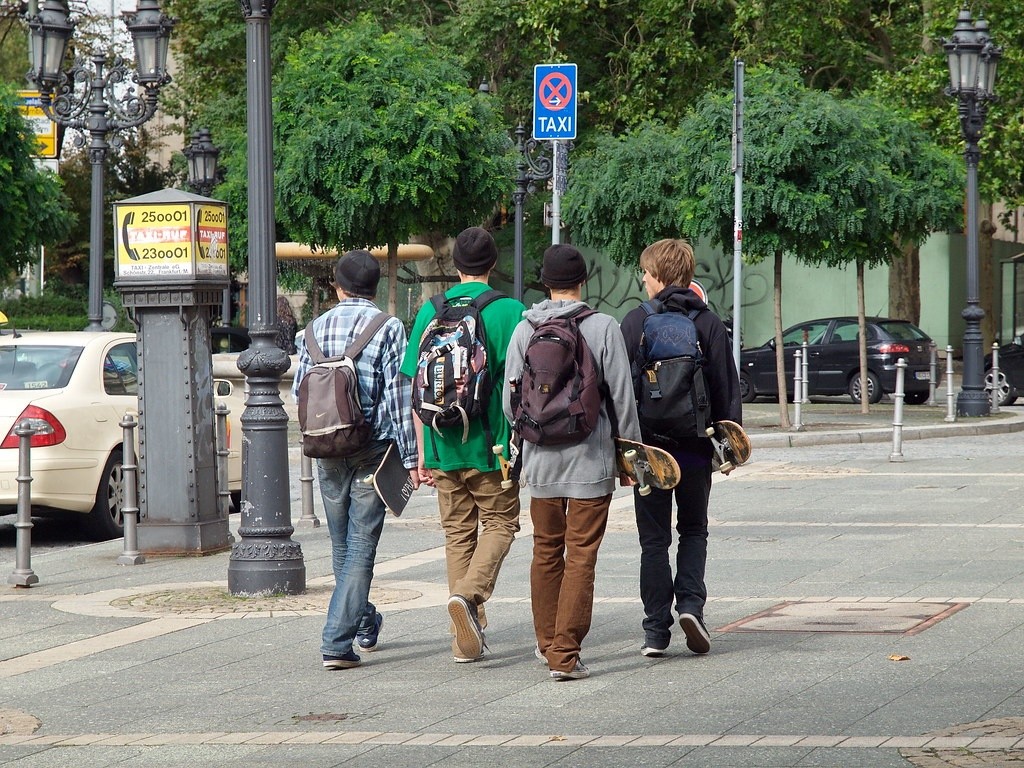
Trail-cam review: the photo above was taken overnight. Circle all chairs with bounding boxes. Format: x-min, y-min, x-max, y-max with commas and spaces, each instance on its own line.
855, 331, 868, 339
0, 360, 62, 387
832, 334, 842, 342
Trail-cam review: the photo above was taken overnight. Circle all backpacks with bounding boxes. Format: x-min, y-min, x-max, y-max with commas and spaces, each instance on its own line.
630, 298, 710, 448
298, 312, 392, 459
508, 307, 601, 447
410, 291, 506, 427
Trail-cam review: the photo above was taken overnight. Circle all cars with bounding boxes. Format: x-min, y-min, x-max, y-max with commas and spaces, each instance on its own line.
984, 332, 1024, 406
740, 316, 942, 404
0, 311, 245, 540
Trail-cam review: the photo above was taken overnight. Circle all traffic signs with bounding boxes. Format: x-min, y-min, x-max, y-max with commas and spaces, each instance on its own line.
16, 89, 57, 158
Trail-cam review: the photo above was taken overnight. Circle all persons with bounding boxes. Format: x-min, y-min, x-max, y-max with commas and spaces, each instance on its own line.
294, 249, 419, 670
399, 227, 529, 665
621, 238, 743, 655
500, 243, 644, 682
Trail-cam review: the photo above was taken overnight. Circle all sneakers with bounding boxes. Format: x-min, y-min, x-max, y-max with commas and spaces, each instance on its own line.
641, 643, 665, 656
550, 655, 590, 678
356, 612, 382, 651
678, 614, 711, 653
453, 648, 485, 662
448, 595, 492, 659
323, 647, 362, 668
534, 641, 549, 665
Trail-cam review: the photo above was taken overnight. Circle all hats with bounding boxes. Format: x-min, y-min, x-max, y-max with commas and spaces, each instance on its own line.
541, 245, 587, 288
335, 251, 380, 299
453, 227, 498, 275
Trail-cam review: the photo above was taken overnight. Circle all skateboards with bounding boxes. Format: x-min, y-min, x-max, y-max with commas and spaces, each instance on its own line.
363, 438, 416, 519
704, 418, 753, 477
614, 436, 683, 498
491, 428, 524, 490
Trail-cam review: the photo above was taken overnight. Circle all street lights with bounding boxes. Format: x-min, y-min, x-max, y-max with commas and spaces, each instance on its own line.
182, 127, 231, 325
27, 0, 176, 332
477, 75, 553, 302
942, 2, 1003, 416
